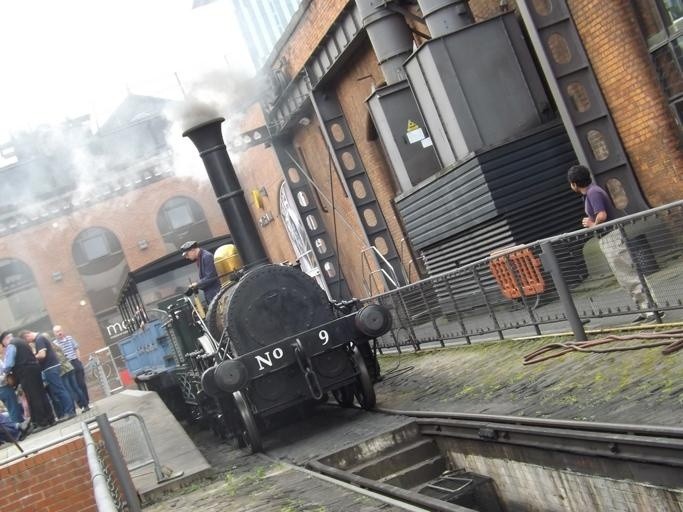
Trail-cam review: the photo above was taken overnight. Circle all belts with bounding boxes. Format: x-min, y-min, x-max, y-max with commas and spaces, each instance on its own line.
597, 230, 613, 239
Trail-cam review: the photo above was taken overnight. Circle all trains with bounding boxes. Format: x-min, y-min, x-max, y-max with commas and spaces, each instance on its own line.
116, 118, 393, 454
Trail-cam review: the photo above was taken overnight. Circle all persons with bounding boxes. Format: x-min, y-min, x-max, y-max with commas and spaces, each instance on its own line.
179, 238, 222, 307
1, 328, 90, 447
566, 163, 667, 327
50, 323, 93, 410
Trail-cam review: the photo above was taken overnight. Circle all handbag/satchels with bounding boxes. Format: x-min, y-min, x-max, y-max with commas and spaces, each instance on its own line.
6, 374, 17, 386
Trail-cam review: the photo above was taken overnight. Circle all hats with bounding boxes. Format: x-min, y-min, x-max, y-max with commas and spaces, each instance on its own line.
180, 241, 200, 257
0, 329, 11, 343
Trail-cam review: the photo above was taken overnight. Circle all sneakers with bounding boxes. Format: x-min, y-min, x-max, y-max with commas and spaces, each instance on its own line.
629, 310, 666, 326
17, 416, 34, 442
31, 405, 94, 434
